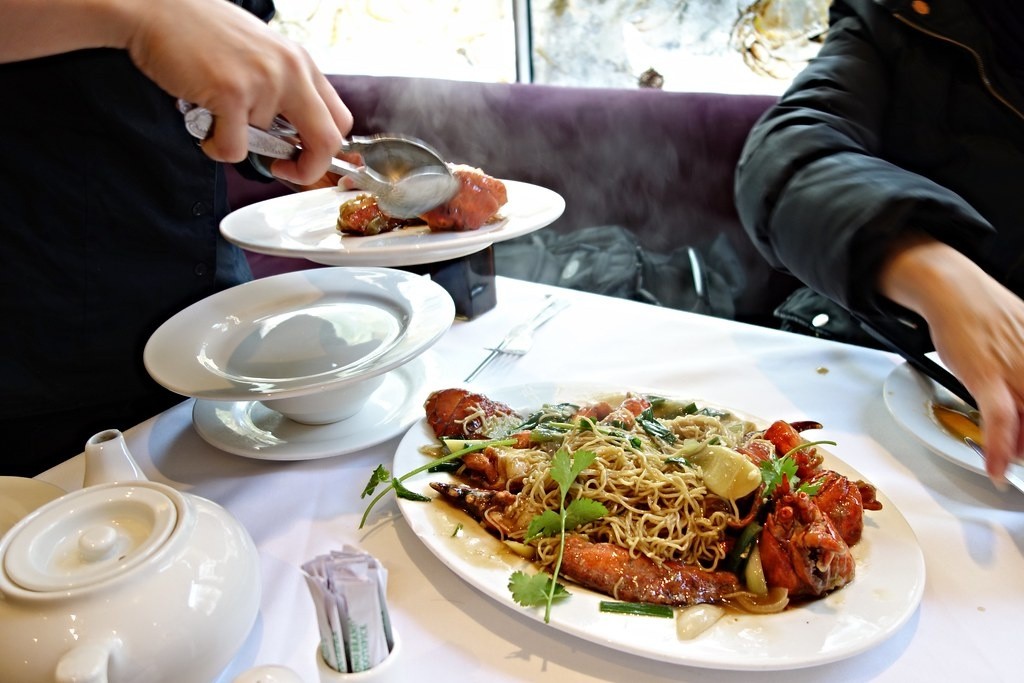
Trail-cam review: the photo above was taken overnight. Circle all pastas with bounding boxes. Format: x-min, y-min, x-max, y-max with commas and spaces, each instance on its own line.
460, 403, 739, 571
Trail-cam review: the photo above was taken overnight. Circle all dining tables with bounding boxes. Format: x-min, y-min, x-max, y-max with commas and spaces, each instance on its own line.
0, 274, 1024, 683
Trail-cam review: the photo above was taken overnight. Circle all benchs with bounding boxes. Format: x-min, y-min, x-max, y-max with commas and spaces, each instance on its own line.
224, 74, 802, 334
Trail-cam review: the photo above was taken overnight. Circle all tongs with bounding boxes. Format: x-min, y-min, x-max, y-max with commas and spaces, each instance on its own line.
179, 100, 458, 219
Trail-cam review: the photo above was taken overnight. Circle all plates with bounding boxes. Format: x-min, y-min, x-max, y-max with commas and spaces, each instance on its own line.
146, 265, 456, 403
220, 177, 567, 266
883, 351, 1024, 487
189, 362, 452, 464
393, 382, 926, 669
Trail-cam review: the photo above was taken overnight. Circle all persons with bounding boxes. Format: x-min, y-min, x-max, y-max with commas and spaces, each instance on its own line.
0, 0, 354, 479
735, 0, 1024, 479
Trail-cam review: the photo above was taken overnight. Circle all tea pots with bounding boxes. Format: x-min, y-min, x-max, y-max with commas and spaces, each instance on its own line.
0, 428, 261, 683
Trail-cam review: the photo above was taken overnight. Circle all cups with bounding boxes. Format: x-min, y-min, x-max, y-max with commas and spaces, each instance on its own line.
317, 629, 401, 683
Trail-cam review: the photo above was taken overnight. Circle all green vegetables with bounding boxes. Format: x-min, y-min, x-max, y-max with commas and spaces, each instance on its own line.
509, 448, 610, 621
358, 440, 517, 531
754, 441, 837, 499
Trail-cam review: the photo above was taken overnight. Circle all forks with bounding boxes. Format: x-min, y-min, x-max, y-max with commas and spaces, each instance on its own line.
484, 298, 571, 354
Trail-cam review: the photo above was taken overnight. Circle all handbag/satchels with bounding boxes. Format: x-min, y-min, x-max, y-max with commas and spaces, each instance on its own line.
642, 238, 775, 320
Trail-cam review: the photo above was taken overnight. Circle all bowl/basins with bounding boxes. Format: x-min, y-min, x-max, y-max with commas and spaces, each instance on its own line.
259, 375, 384, 425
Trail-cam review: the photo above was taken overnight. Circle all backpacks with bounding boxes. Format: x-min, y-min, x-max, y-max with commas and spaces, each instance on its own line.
494, 225, 662, 306
